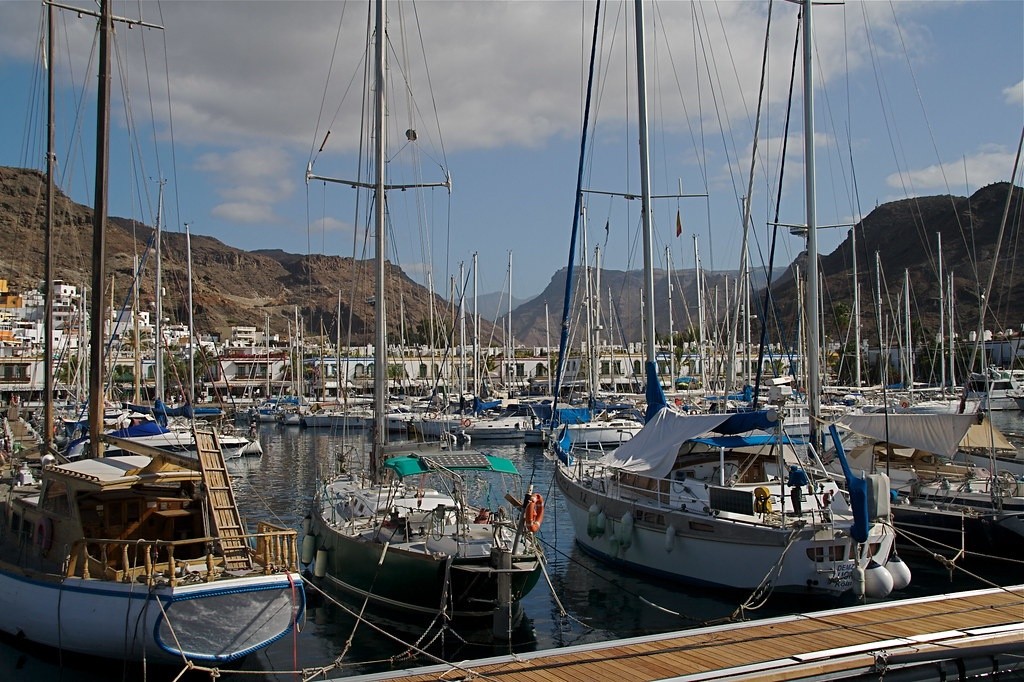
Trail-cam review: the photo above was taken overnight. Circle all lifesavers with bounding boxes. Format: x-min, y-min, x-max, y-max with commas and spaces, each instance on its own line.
34, 517, 52, 556
221, 424, 233, 435
525, 493, 544, 531
464, 419, 471, 427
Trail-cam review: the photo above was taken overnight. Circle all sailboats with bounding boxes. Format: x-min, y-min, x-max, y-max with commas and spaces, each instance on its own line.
1, 0, 1023, 676
